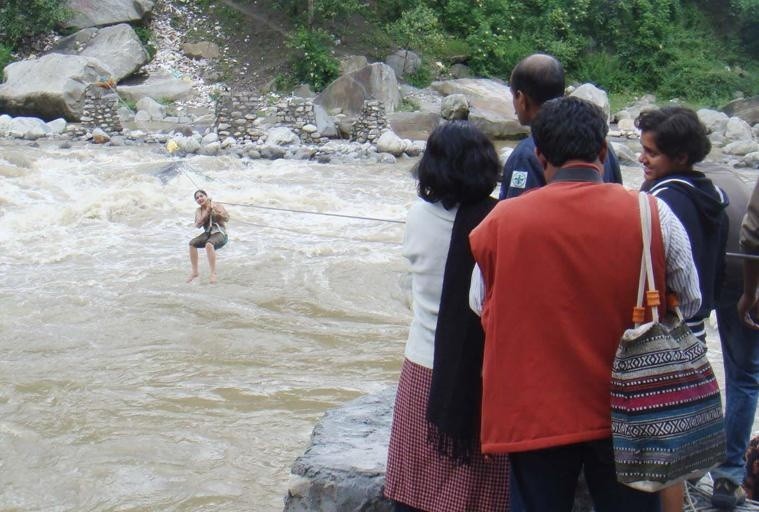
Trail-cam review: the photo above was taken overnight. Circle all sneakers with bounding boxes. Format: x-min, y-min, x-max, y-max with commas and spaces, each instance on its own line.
710, 475, 748, 509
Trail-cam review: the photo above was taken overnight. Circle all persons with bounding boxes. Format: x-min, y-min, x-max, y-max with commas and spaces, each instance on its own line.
735, 177, 758, 335
494, 52, 627, 207
688, 164, 758, 511
461, 95, 706, 511
632, 104, 733, 511
186, 189, 231, 283
380, 115, 517, 510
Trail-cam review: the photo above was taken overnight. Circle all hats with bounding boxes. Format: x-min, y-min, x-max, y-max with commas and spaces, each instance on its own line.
440, 94, 470, 121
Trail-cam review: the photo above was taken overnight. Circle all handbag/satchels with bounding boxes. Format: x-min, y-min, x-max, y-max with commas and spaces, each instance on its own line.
609, 318, 728, 494
